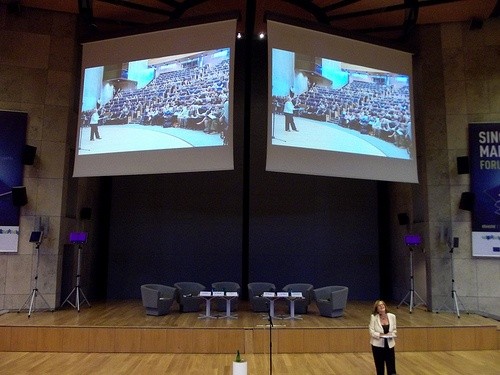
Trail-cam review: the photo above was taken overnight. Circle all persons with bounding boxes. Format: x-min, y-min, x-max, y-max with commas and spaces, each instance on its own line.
369, 300, 397, 375
81, 59, 229, 145
272, 80, 411, 150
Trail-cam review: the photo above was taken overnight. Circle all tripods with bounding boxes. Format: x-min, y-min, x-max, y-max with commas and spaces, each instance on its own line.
435, 249, 470, 318
397, 244, 431, 312
16, 242, 56, 318
59, 244, 91, 312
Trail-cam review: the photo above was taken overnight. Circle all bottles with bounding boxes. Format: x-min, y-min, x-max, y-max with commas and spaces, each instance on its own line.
236, 350, 241, 362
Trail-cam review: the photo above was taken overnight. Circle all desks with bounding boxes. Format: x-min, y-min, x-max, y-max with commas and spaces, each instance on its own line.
260, 292, 306, 321
192, 292, 240, 320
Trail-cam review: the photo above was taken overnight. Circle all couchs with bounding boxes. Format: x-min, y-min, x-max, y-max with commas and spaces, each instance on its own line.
248, 282, 276, 312
312, 286, 349, 318
212, 282, 240, 311
174, 282, 206, 313
141, 284, 176, 315
282, 283, 314, 315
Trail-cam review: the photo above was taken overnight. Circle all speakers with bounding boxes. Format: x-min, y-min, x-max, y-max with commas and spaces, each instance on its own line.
11, 186, 27, 207
468, 17, 484, 30
457, 156, 471, 174
459, 192, 476, 210
21, 144, 37, 165
